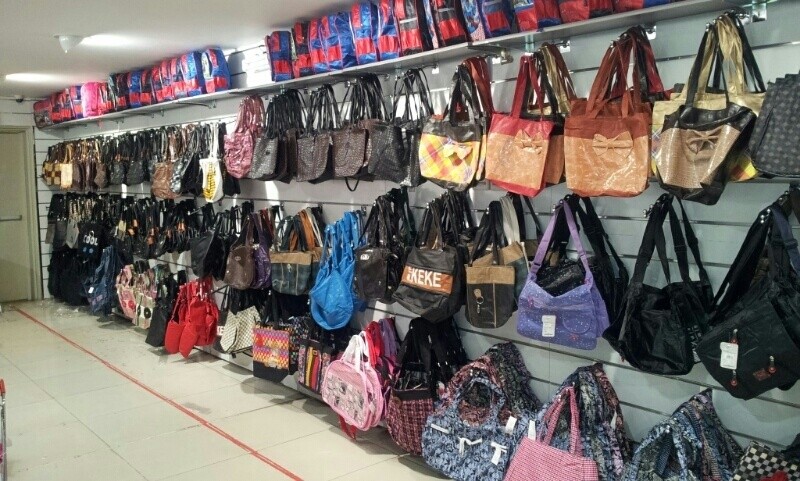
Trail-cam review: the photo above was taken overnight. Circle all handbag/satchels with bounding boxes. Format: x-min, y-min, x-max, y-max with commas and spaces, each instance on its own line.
32, 1, 799, 481
